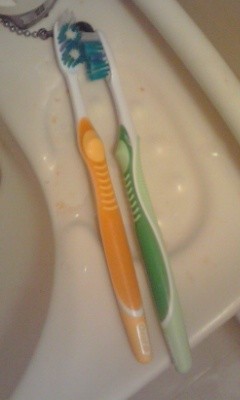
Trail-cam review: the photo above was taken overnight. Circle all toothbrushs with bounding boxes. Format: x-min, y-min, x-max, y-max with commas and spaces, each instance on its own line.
53, 9, 153, 364
79, 29, 192, 373
